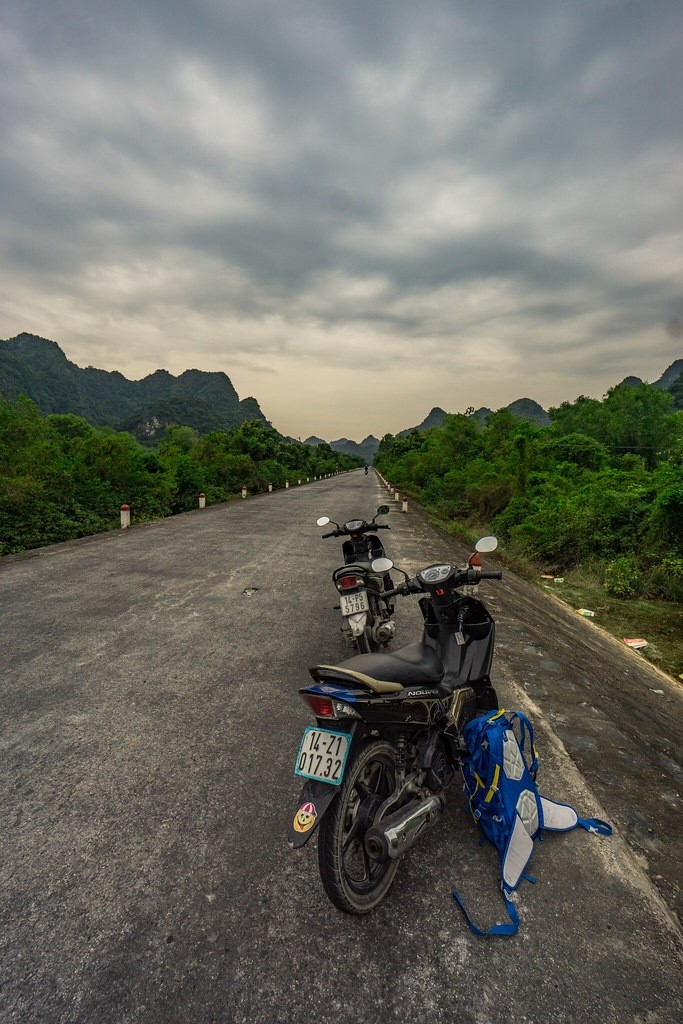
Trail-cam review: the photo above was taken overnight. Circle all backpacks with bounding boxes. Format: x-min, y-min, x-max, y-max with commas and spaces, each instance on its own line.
452, 707, 612, 936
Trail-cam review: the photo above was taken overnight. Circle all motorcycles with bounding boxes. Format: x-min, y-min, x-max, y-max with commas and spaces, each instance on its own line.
364, 467, 368, 474
286, 536, 506, 917
316, 504, 399, 654
